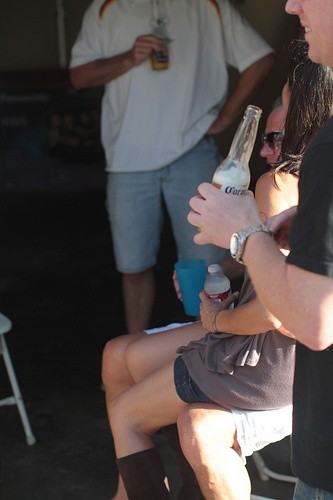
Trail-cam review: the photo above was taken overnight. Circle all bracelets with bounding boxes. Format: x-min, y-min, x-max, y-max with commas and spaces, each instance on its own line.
212, 309, 223, 332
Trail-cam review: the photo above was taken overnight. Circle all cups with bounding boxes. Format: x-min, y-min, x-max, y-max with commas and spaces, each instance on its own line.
174, 259, 207, 316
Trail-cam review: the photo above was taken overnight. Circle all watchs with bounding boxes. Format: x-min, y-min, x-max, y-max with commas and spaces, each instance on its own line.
230, 224, 273, 264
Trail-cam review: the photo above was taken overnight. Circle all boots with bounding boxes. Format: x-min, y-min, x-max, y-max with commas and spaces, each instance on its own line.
163, 425, 202, 500
115, 446, 172, 500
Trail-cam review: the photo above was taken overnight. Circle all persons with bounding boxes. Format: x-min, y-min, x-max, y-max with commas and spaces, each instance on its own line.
68, 0, 275, 333
187, 1, 333, 500
101, 54, 333, 500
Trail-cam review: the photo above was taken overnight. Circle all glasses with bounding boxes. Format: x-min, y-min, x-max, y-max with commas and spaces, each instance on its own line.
261, 131, 285, 151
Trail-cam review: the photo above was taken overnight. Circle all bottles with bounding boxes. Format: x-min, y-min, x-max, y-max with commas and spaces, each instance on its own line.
149, 0, 170, 70
198, 104, 262, 233
204, 264, 235, 339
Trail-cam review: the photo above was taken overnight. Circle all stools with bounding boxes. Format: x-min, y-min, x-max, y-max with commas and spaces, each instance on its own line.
0, 313, 36, 445
